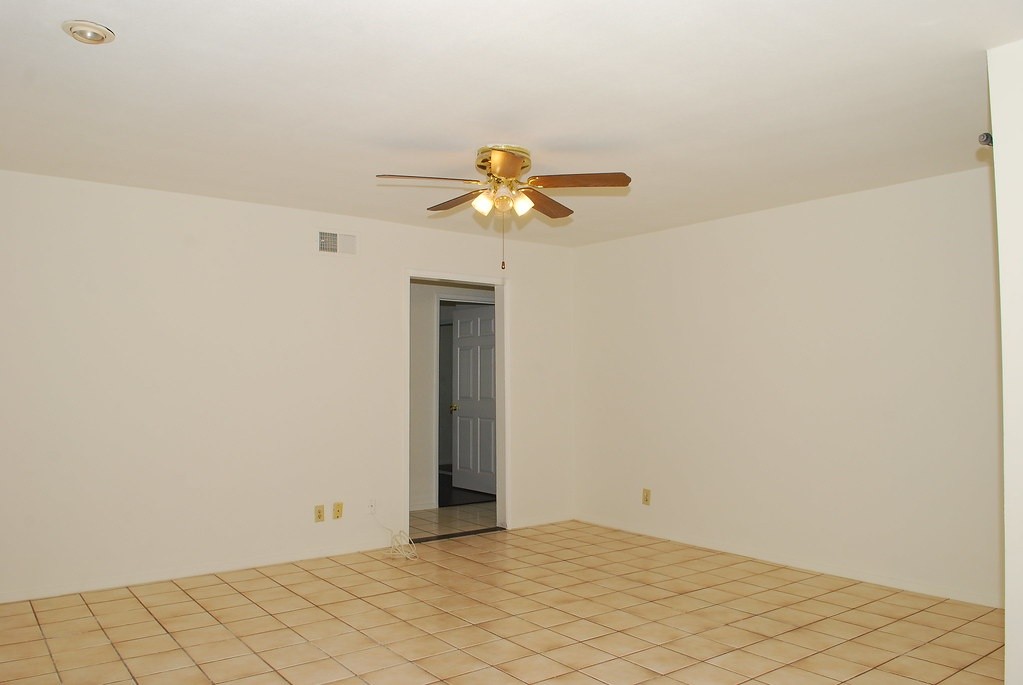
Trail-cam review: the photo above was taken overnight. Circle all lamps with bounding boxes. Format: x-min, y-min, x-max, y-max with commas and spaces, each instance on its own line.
472, 182, 534, 269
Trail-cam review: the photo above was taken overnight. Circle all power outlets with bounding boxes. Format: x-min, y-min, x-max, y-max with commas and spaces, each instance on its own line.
333, 503, 343, 519
314, 505, 324, 521
642, 489, 651, 505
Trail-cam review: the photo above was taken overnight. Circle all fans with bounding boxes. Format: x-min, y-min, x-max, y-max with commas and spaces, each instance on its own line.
376, 146, 631, 218
